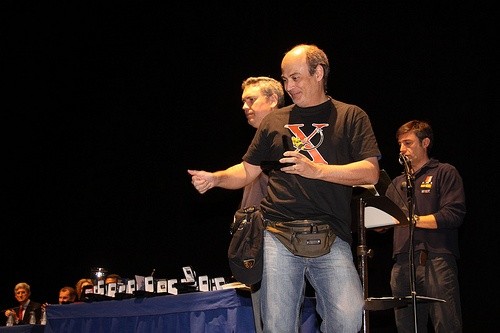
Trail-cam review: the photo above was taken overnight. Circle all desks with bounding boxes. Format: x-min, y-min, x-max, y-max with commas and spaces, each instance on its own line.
45, 287, 257, 333
0, 324, 47, 333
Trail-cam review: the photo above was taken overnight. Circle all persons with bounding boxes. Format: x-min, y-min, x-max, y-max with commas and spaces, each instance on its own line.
375, 121, 465, 333
41, 286, 78, 313
187, 44, 382, 333
76, 278, 93, 299
104, 274, 119, 284
239, 76, 322, 333
4, 282, 43, 326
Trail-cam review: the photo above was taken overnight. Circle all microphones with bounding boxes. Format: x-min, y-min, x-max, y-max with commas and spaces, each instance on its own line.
397, 153, 415, 177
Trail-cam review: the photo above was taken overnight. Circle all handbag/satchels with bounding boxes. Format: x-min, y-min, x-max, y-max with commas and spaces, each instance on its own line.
262, 216, 338, 258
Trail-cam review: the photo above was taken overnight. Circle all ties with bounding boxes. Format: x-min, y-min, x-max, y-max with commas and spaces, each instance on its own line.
18, 305, 23, 320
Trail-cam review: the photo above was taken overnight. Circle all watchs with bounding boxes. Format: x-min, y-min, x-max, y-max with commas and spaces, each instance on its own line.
414, 214, 419, 223
